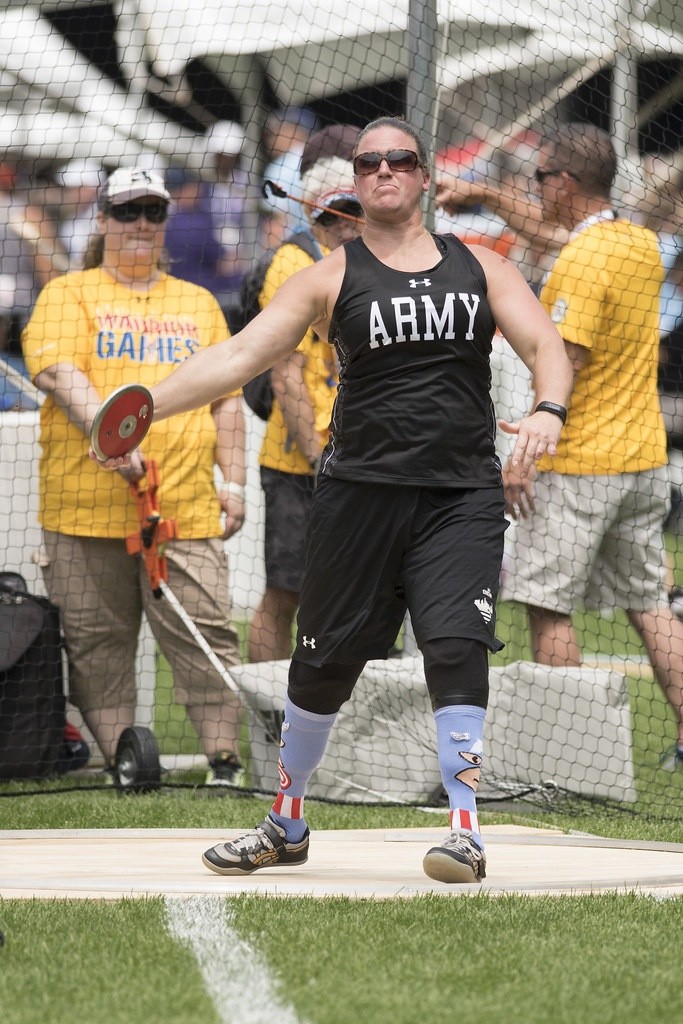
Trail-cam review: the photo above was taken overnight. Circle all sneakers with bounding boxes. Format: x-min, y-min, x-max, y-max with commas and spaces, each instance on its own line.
202, 815, 310, 875
423, 828, 487, 882
205, 760, 246, 786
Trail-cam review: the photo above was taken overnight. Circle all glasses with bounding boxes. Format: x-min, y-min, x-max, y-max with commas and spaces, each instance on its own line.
109, 203, 168, 223
353, 149, 424, 176
317, 201, 361, 225
535, 168, 580, 186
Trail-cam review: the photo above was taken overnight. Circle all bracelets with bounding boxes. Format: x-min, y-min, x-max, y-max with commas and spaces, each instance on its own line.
221, 482, 245, 499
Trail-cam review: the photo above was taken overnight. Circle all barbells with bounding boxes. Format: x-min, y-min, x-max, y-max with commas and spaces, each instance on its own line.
115, 728, 211, 796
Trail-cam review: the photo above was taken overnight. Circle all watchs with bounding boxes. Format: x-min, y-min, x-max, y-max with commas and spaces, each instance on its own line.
534, 401, 567, 426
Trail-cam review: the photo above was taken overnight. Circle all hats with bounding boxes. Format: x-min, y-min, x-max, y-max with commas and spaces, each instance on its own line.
301, 157, 364, 224
97, 167, 171, 211
199, 120, 246, 156
55, 159, 104, 188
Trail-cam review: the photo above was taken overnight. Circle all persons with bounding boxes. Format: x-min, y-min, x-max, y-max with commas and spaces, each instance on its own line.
88, 118, 578, 883
0, 118, 683, 795
